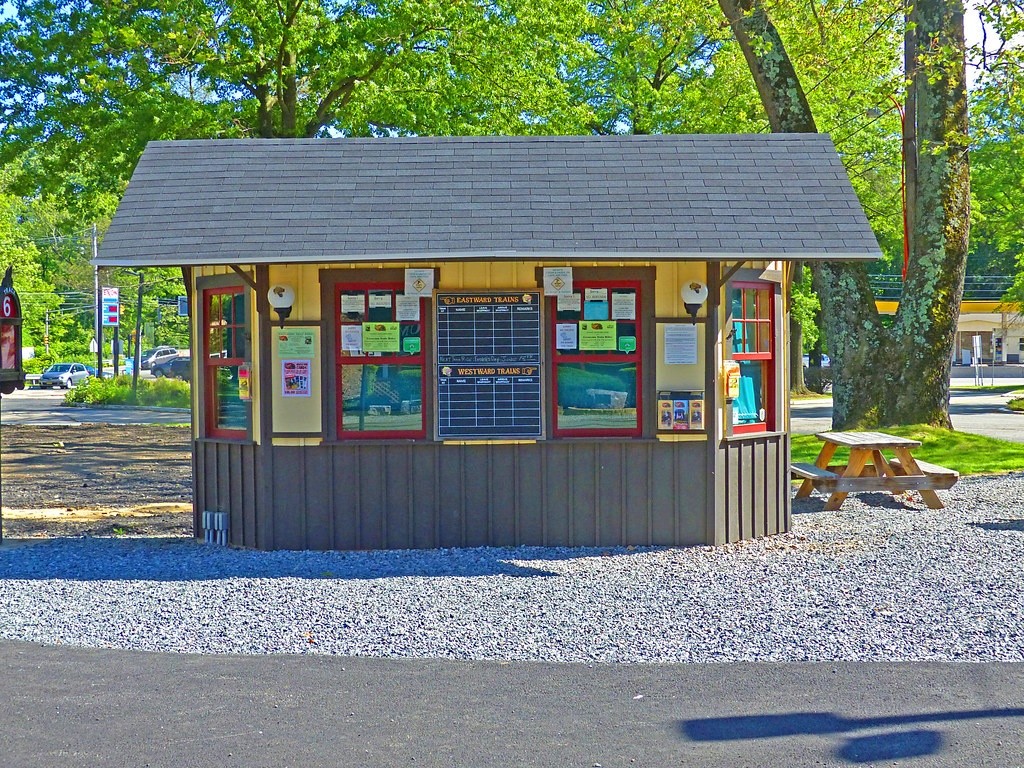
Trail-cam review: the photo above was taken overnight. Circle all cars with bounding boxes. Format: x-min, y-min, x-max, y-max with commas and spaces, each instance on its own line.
40, 363, 89, 389
141, 347, 179, 370
170, 361, 190, 381
802, 353, 831, 370
150, 356, 189, 378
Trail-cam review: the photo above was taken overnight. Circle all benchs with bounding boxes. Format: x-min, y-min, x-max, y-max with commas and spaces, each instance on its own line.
791, 462, 839, 482
890, 456, 960, 479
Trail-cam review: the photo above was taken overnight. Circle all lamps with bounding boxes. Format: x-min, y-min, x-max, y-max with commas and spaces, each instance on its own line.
268, 284, 296, 329
681, 280, 708, 325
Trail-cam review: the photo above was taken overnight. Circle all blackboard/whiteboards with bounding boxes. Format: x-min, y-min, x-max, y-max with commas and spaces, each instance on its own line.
431, 287, 546, 441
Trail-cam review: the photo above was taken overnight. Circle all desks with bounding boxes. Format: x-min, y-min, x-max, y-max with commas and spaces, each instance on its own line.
791, 432, 958, 510
